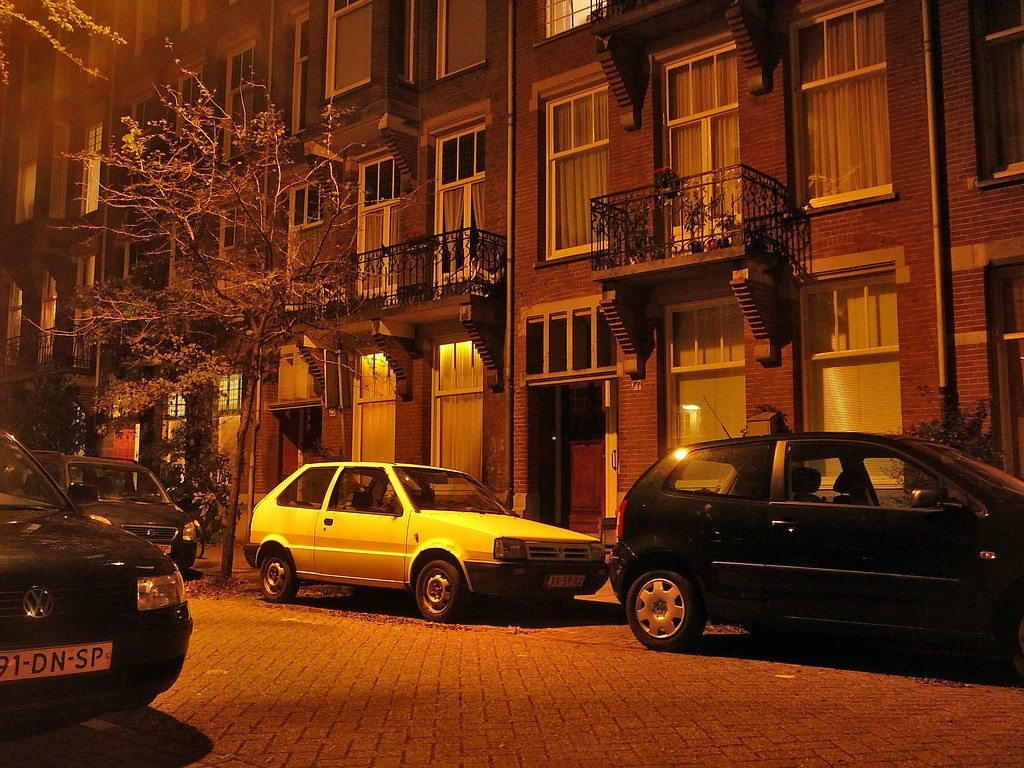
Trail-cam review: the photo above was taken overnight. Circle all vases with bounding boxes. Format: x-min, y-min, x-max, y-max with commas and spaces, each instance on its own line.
408, 233, 439, 254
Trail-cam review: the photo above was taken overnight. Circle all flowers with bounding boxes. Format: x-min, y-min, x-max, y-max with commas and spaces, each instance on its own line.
655, 166, 678, 187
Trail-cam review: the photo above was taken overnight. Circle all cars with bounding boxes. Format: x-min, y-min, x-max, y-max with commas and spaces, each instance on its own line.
608, 431, 1024, 686
0, 431, 193, 713
244, 461, 607, 622
5, 452, 197, 575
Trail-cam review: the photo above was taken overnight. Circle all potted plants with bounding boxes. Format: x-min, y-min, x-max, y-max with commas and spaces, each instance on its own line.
683, 181, 743, 254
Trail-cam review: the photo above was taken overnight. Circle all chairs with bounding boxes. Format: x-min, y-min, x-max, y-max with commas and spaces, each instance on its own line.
351, 485, 383, 513
96, 477, 113, 496
421, 487, 436, 503
24, 474, 44, 495
832, 472, 868, 505
790, 467, 822, 502
